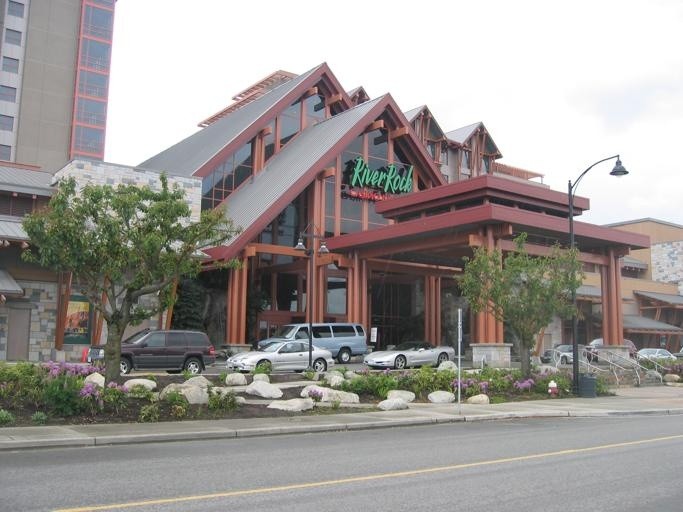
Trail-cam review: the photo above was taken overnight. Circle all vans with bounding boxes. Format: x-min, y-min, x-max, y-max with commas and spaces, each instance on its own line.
257, 323, 368, 364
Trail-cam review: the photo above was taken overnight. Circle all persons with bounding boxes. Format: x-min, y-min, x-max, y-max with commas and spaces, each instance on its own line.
74, 311, 88, 337
65, 314, 74, 332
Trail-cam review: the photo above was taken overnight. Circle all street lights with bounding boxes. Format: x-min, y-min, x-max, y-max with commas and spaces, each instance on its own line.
292, 218, 331, 368
567, 153, 630, 396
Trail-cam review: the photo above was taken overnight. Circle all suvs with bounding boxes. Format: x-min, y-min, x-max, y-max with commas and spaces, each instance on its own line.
87, 328, 216, 377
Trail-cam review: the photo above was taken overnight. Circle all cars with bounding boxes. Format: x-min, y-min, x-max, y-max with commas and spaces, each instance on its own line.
540, 337, 683, 365
228, 340, 336, 376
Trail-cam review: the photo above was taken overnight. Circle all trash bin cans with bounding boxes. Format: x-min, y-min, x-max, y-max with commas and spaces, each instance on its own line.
578, 372, 596, 397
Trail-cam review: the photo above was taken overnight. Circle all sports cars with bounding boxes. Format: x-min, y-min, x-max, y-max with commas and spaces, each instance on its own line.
365, 340, 455, 369
